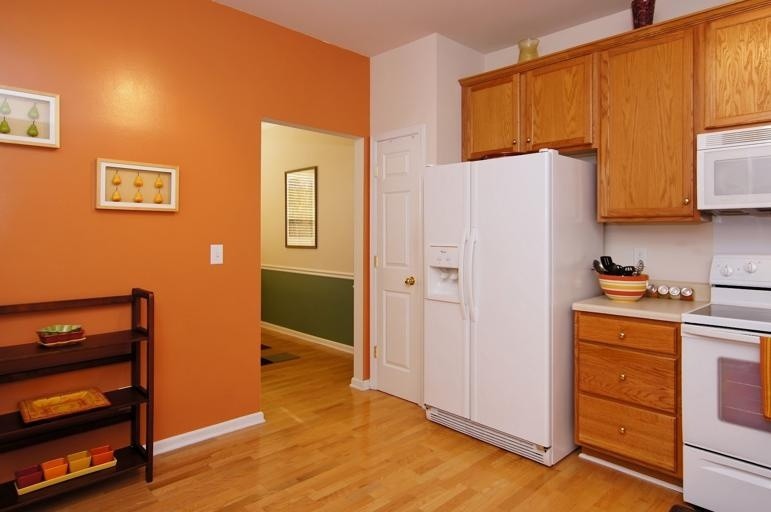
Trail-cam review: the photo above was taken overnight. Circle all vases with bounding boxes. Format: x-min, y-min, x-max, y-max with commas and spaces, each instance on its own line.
518, 37, 538, 62
630, 0, 654, 27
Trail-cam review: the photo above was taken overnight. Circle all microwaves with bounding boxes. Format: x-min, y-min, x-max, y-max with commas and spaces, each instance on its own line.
695, 124, 771, 219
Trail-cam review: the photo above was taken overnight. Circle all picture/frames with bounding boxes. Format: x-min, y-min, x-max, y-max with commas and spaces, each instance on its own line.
95, 158, 180, 212
285, 167, 318, 249
0, 86, 60, 149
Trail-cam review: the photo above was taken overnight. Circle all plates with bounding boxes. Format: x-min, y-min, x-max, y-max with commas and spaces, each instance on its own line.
14, 456, 118, 495
36, 323, 87, 348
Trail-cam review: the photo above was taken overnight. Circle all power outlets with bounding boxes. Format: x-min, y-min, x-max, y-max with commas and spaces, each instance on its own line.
210, 244, 224, 264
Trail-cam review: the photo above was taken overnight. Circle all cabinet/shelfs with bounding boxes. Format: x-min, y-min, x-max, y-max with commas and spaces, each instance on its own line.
457, 40, 598, 160
596, 14, 710, 225
571, 295, 710, 485
1, 287, 156, 509
699, 0, 771, 130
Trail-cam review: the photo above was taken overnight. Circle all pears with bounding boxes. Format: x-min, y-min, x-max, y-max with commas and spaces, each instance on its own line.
0, 97, 11, 114
112, 186, 121, 201
27, 121, 38, 137
154, 189, 163, 203
28, 102, 39, 119
0, 116, 10, 133
134, 188, 143, 202
112, 169, 122, 185
134, 170, 144, 187
154, 173, 163, 188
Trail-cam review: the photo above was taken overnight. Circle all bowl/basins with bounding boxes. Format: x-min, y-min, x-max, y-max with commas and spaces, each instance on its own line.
597, 274, 650, 302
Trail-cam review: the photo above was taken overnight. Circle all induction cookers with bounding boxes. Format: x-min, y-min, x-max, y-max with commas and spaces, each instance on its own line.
680, 254, 771, 334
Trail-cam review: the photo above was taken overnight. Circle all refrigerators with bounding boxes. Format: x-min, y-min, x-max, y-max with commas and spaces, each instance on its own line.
423, 152, 605, 467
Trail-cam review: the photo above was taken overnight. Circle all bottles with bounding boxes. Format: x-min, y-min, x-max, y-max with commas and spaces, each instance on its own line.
680, 287, 694, 300
657, 285, 669, 297
669, 286, 680, 299
